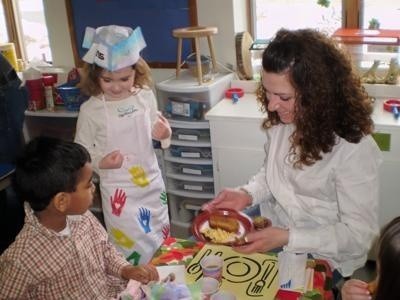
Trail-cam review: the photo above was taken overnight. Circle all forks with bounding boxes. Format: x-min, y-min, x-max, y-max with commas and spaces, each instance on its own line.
251, 261, 275, 293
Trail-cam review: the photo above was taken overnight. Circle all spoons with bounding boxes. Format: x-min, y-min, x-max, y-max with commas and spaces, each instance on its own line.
187, 249, 213, 275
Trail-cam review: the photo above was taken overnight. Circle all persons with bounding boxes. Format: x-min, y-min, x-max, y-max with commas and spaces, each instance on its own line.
73, 24, 173, 267
0, 52, 30, 166
341, 214, 400, 300
0, 135, 161, 300
199, 26, 382, 278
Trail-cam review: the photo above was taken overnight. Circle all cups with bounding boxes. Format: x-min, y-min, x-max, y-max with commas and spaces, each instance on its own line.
209, 292, 236, 300
196, 277, 219, 300
200, 256, 224, 283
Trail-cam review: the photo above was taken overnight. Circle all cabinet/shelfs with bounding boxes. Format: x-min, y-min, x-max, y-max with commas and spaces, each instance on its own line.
205, 80, 399, 262
21, 110, 103, 214
155, 72, 233, 239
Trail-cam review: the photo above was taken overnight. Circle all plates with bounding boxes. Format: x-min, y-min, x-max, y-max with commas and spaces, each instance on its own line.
221, 256, 261, 283
191, 207, 254, 247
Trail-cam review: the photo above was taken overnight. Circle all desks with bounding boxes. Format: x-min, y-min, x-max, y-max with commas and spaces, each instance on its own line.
117, 236, 334, 300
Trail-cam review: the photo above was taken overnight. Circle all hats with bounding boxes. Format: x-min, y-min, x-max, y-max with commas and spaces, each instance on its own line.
82, 26, 148, 73
